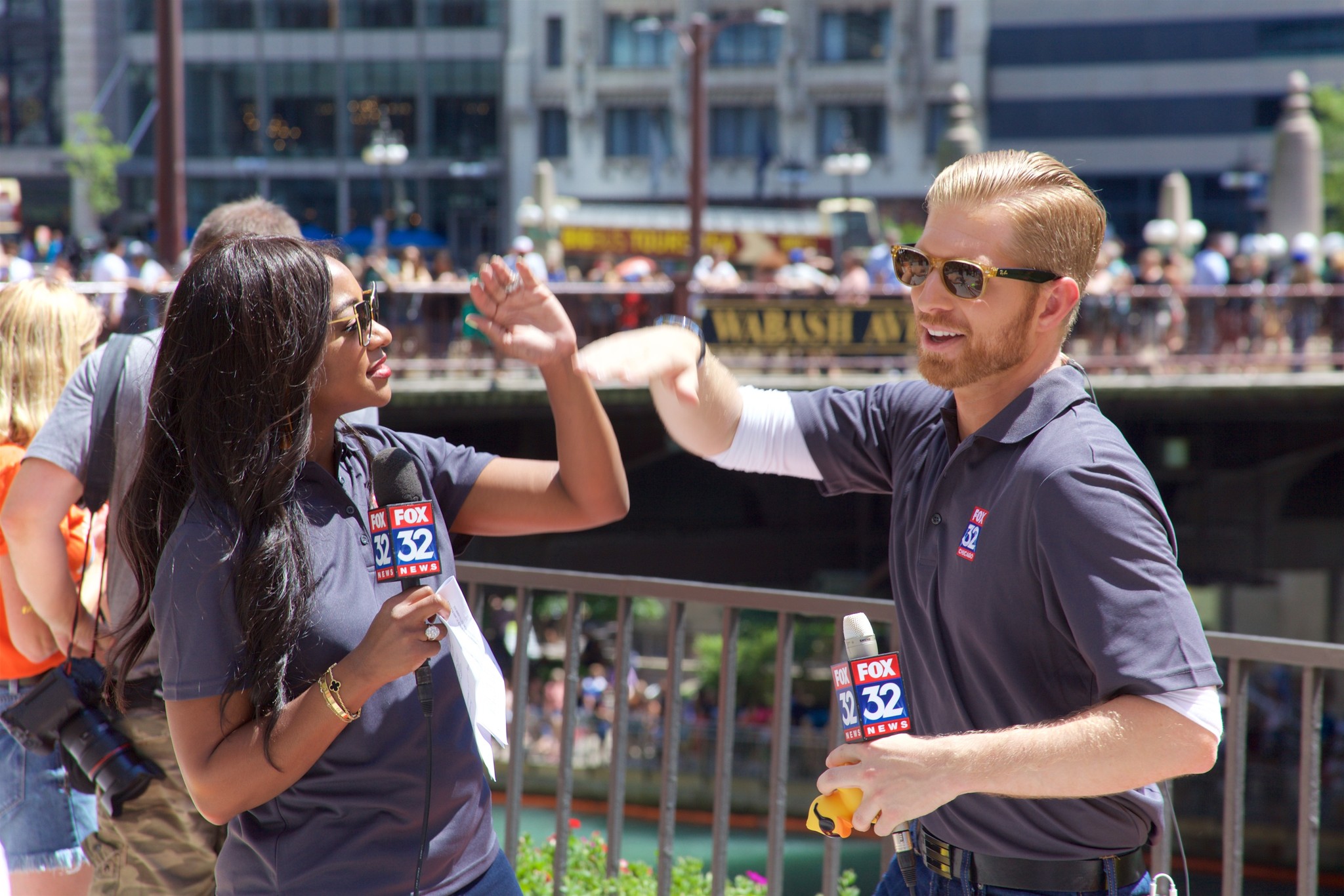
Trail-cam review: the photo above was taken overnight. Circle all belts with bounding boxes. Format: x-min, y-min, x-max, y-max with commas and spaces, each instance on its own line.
105, 674, 164, 710
915, 820, 1147, 893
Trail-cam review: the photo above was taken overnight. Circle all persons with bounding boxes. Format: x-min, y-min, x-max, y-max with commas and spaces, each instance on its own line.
572, 148, 1221, 896
1, 199, 299, 896
501, 661, 830, 760
1, 224, 1344, 377
116, 236, 631, 896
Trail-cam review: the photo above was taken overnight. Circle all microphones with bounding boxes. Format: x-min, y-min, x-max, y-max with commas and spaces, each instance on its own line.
829, 611, 917, 888
370, 446, 441, 717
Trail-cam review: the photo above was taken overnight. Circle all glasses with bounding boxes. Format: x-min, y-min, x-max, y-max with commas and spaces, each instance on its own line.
328, 280, 380, 347
889, 242, 1062, 301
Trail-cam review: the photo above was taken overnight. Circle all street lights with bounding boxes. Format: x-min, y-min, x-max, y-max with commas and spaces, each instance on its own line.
628, 4, 790, 268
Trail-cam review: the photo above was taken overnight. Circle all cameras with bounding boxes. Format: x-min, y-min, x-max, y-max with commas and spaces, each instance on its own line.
0, 656, 167, 819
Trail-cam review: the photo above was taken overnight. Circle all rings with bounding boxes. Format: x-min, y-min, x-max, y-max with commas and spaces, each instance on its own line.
425, 624, 440, 641
504, 272, 521, 294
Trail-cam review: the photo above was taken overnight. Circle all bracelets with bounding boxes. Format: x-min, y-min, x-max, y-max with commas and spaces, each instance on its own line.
654, 314, 706, 367
318, 662, 361, 723
